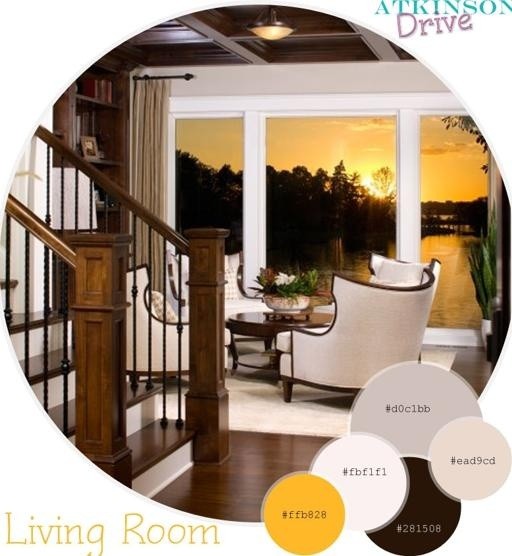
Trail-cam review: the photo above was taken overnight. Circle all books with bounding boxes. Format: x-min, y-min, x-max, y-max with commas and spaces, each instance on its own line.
83, 78, 98, 100
106, 81, 112, 103
96, 79, 108, 103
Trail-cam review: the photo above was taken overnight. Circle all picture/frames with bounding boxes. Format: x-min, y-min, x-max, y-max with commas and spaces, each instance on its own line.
80, 135, 100, 161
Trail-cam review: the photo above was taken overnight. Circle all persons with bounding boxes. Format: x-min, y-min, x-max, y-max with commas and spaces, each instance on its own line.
85, 140, 96, 156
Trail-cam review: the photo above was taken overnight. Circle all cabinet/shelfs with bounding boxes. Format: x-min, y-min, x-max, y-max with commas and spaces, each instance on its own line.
53, 66, 131, 235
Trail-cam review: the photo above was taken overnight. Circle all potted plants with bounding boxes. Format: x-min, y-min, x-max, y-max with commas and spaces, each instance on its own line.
467, 198, 498, 355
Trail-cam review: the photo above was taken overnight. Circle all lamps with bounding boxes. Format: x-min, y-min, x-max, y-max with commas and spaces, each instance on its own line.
246, 5, 296, 42
